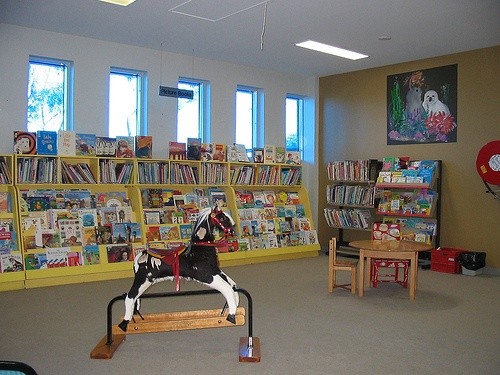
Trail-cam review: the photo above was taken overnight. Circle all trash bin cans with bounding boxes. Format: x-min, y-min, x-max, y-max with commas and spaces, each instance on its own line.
460, 252, 487, 275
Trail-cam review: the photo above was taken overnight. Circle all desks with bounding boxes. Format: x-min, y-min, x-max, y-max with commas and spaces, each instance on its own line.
348, 239, 432, 300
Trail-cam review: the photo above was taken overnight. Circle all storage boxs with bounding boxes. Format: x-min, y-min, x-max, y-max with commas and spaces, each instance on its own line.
430, 248, 468, 274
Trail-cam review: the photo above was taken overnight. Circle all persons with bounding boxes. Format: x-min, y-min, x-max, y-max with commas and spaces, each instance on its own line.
119, 251, 130, 263
116, 139, 135, 158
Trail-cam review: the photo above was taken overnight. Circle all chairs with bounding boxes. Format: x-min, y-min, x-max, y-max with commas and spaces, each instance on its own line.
371, 223, 409, 288
327, 238, 358, 294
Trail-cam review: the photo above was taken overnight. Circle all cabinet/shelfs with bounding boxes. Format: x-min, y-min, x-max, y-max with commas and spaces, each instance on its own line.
0, 153, 323, 291
327, 159, 441, 270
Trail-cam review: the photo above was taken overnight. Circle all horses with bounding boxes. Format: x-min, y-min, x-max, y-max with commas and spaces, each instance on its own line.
118, 205, 239, 333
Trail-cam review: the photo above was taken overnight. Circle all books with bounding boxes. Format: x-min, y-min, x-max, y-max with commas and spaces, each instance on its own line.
324, 159, 377, 229
0, 130, 318, 272
